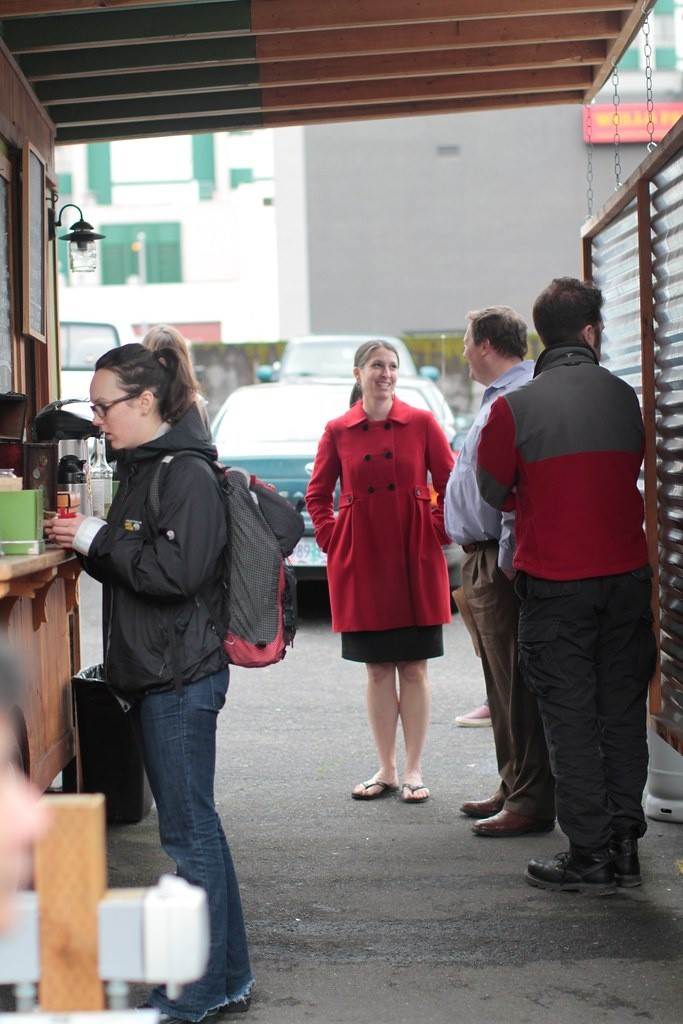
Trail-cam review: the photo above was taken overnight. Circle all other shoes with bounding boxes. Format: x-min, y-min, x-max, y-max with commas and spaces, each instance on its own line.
455, 705, 492, 727
136, 987, 252, 1024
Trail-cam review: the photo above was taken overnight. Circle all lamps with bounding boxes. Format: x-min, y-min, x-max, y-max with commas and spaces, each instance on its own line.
49, 204, 106, 273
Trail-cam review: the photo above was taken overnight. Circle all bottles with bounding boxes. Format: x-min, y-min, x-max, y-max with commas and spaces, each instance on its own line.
0, 468, 18, 479
58, 431, 113, 521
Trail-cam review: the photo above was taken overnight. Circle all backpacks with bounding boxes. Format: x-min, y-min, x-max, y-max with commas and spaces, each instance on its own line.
151, 450, 305, 668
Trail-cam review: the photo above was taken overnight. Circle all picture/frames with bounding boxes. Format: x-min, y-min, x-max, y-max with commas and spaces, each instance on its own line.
19, 137, 48, 347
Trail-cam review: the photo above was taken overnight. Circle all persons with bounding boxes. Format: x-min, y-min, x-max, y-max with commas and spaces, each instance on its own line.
43, 342, 254, 1024
141, 326, 213, 440
474, 277, 659, 897
452, 698, 493, 727
304, 340, 456, 804
444, 306, 555, 836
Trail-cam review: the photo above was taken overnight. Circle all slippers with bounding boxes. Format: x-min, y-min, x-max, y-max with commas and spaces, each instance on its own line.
351, 781, 399, 801
401, 784, 429, 803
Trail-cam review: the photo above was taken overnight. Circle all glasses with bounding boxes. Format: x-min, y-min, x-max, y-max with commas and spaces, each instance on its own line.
90, 391, 159, 418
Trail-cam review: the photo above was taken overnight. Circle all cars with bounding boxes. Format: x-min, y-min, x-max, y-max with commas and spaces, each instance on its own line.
260, 337, 445, 383
57, 323, 134, 517
200, 372, 472, 611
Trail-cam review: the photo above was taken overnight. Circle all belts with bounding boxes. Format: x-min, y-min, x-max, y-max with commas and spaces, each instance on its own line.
462, 539, 498, 554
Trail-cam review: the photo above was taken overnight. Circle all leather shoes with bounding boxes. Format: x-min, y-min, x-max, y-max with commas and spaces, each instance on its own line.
459, 793, 555, 837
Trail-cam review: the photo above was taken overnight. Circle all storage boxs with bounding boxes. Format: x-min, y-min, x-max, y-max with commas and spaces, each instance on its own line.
0, 485, 47, 555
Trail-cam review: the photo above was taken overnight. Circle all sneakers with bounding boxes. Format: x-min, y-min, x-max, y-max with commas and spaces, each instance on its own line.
523, 838, 642, 896
